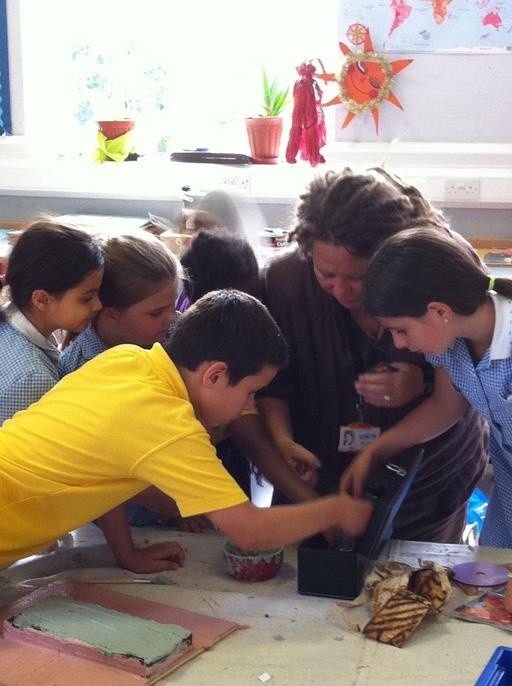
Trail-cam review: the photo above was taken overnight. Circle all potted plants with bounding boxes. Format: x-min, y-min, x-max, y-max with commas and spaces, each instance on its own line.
244, 62, 290, 165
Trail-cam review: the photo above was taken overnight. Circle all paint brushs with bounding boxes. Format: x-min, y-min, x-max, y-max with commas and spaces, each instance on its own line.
81, 575, 175, 583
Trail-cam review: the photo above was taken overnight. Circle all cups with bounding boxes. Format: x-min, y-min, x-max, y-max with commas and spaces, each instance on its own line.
96, 120, 134, 139
257, 227, 289, 248
223, 538, 283, 580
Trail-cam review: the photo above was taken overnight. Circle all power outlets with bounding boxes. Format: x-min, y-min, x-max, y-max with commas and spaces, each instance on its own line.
442, 178, 481, 201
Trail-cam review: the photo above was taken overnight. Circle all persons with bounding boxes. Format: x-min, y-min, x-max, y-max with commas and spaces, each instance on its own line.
285, 57, 328, 166
56, 229, 203, 535
252, 164, 490, 548
342, 431, 356, 446
169, 227, 351, 551
0, 288, 374, 573
1, 221, 105, 426
337, 227, 512, 548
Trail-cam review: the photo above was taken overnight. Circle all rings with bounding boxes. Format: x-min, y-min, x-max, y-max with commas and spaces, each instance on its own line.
383, 394, 391, 408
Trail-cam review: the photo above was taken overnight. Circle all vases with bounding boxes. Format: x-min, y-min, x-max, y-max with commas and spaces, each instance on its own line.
96, 121, 134, 158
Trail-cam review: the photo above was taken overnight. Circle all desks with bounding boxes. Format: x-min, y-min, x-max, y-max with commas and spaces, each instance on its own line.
1, 522, 512, 686
